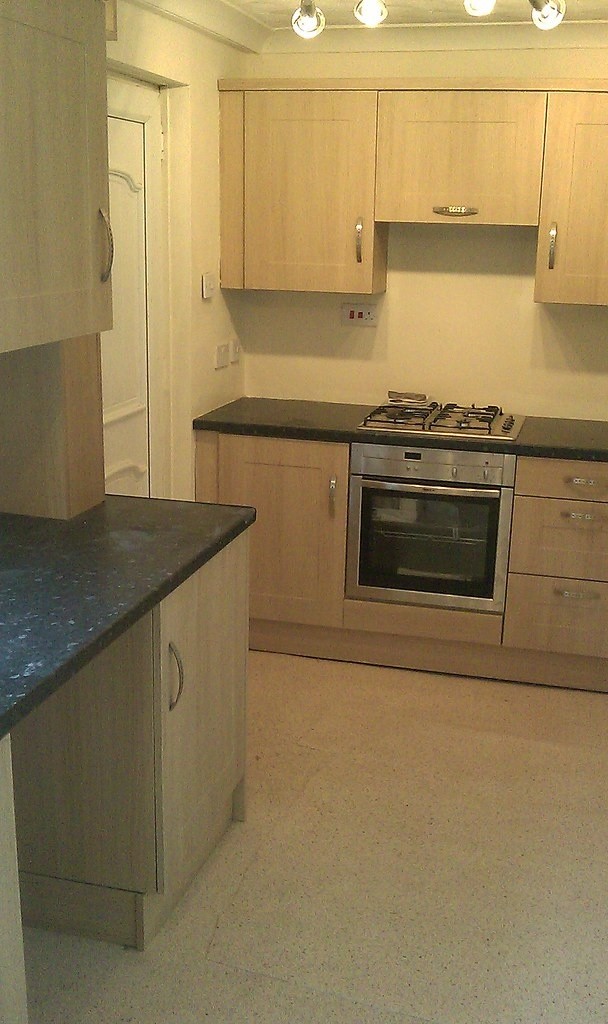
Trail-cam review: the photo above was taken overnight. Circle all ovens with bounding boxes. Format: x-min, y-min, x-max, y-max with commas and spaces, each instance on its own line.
343, 443, 517, 646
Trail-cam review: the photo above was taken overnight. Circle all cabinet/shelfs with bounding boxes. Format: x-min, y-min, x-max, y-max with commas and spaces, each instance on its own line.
217, 76, 608, 306
192, 429, 607, 692
9, 524, 248, 952
0, 0, 114, 354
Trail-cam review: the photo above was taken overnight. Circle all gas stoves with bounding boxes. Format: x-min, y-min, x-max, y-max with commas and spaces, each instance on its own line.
356, 390, 527, 441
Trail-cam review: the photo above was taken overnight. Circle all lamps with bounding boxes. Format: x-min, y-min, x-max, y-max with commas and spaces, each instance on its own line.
291, 0, 569, 39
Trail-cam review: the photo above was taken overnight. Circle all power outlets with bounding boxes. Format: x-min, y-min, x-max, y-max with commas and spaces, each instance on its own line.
341, 302, 380, 329
